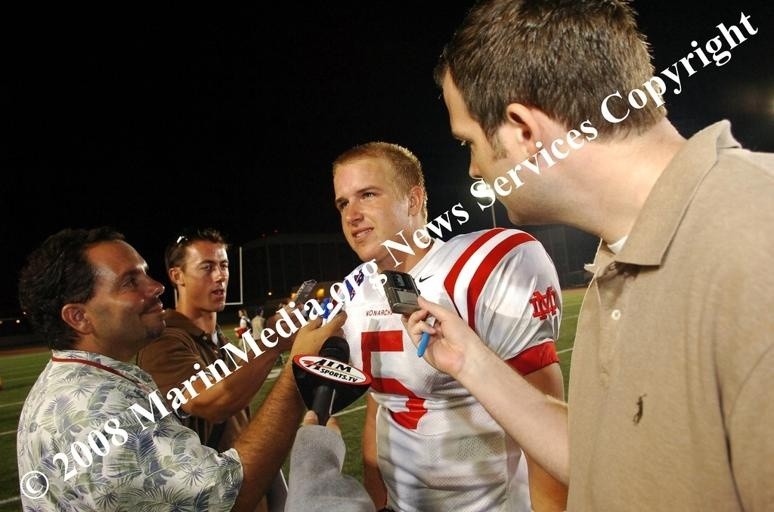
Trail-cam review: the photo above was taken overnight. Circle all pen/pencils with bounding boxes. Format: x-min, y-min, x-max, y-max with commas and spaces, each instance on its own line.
418, 316, 437, 358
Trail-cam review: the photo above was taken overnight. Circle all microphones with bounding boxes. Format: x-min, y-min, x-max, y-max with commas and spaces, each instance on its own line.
292, 336, 372, 427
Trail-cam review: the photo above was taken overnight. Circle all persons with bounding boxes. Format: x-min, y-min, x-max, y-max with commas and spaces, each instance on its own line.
401, 0, 774, 512
283, 408, 376, 512
137, 228, 305, 512
235, 310, 253, 354
251, 307, 267, 340
330, 142, 568, 512
17, 228, 347, 512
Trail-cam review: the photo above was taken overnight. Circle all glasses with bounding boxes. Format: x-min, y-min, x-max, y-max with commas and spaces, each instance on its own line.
167, 229, 193, 264
